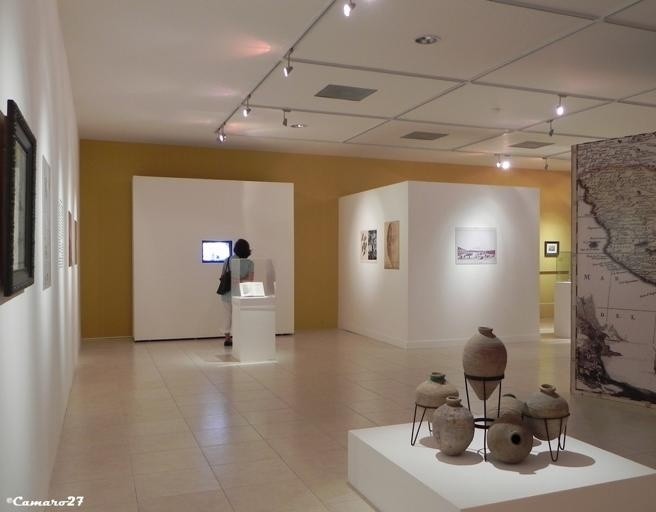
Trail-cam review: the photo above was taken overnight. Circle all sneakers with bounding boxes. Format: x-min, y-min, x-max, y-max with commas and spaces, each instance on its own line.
224, 337, 232, 345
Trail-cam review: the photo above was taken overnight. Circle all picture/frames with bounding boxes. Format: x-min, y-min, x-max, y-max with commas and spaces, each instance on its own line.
455, 224, 498, 267
545, 240, 560, 257
2, 93, 38, 295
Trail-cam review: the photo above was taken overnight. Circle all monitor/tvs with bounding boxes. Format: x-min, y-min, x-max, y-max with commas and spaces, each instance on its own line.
202, 240, 232, 264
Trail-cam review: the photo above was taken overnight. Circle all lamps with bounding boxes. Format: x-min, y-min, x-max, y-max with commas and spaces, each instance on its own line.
555, 93, 568, 117
544, 120, 555, 139
216, 1, 362, 145
492, 153, 551, 173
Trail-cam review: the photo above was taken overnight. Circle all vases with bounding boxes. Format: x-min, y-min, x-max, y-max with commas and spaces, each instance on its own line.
412, 322, 572, 466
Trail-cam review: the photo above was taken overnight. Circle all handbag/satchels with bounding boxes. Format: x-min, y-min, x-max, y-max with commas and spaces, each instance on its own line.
217, 271, 231, 294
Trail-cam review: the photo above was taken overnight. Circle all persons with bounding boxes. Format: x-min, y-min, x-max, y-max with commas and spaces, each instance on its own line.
220, 239, 254, 346
